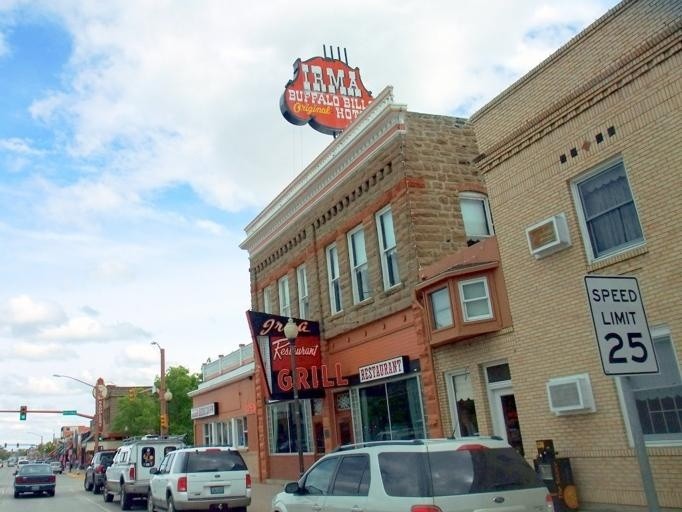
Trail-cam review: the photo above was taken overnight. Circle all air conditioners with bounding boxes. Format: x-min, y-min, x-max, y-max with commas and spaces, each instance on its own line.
545, 372, 597, 417
525, 211, 573, 260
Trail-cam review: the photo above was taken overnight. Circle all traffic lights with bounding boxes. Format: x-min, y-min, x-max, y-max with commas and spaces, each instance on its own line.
20, 406, 27, 420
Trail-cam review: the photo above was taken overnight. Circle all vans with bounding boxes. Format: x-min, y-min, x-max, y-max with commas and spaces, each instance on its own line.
16, 460, 30, 472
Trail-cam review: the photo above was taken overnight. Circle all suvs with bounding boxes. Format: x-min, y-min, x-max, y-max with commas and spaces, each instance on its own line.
84, 451, 116, 493
271, 434, 555, 512
147, 445, 252, 512
102, 434, 191, 510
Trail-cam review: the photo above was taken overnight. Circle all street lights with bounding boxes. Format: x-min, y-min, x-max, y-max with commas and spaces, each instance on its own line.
53, 374, 108, 454
284, 317, 305, 477
152, 340, 172, 435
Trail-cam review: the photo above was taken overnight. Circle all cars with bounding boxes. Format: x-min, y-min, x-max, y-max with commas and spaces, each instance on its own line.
51, 461, 63, 474
13, 464, 56, 497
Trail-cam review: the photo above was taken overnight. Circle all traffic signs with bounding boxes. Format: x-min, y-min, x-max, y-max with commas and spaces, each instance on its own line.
584, 277, 660, 375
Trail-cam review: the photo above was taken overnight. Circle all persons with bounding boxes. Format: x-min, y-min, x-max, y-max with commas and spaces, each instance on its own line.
143, 451, 154, 463
